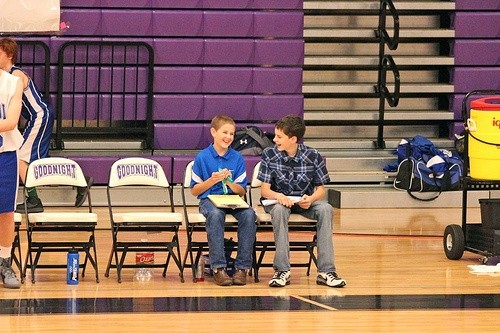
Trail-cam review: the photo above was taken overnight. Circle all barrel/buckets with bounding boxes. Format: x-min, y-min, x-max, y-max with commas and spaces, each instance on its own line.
467, 94, 500, 181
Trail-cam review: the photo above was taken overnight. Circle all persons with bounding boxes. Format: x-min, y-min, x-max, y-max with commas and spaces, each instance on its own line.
256, 115, 346, 287
190, 115, 257, 286
0, 69, 24, 287
0, 38, 54, 212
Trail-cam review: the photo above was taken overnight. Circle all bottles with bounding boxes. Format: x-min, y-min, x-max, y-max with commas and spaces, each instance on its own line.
195, 252, 205, 282
135, 239, 155, 283
67, 246, 80, 285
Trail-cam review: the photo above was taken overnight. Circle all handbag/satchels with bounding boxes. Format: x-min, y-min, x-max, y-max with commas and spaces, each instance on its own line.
231, 126, 274, 152
394, 145, 464, 201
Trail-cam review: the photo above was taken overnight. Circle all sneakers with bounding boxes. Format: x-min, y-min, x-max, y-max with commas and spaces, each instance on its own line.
316, 271, 346, 287
269, 269, 291, 287
0, 257, 21, 288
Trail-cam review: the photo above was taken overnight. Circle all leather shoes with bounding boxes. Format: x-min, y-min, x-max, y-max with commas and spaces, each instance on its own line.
213, 267, 233, 285
233, 269, 247, 285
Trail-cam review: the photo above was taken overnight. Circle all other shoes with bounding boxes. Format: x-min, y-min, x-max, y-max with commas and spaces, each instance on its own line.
15, 196, 44, 213
73, 176, 93, 207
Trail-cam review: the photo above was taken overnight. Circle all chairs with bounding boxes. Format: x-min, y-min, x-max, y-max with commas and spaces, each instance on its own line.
179, 160, 259, 284
104, 157, 186, 285
249, 161, 318, 282
11, 213, 25, 284
23, 157, 99, 284
